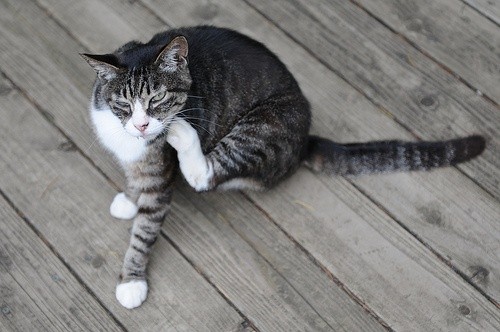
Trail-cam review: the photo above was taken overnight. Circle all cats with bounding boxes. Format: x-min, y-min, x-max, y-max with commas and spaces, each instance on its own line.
77, 24, 486, 310
